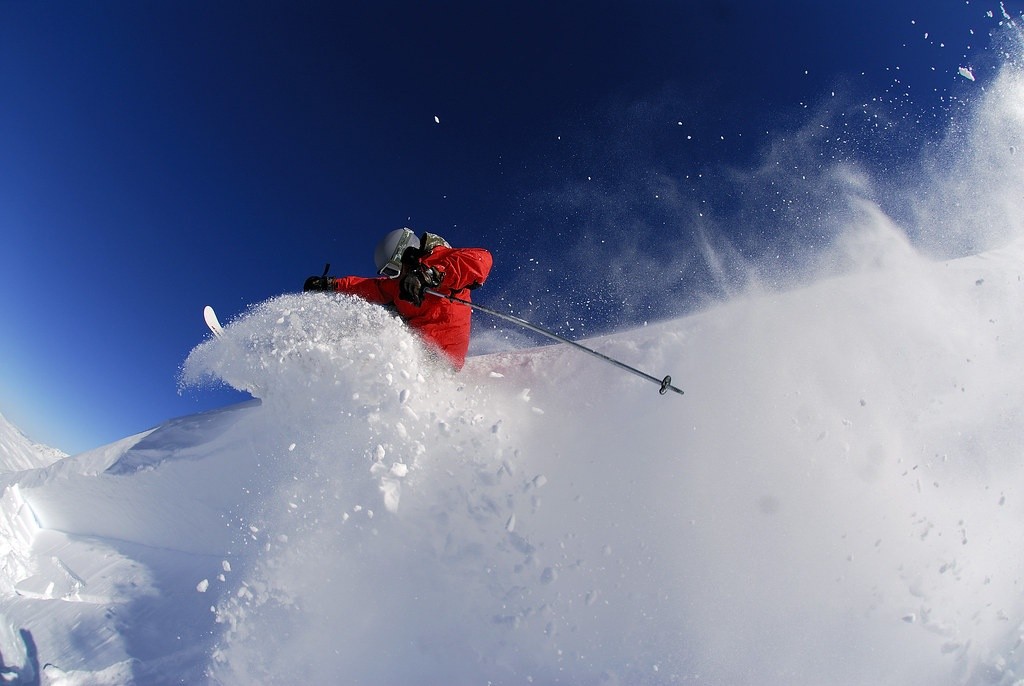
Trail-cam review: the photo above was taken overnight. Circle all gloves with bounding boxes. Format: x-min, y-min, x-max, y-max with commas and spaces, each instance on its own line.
397, 267, 446, 310
303, 276, 336, 292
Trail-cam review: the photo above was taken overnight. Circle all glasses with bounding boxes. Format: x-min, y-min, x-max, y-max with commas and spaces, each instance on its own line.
380, 247, 408, 279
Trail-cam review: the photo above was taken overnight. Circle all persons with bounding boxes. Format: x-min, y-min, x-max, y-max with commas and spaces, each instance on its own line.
301, 226, 494, 378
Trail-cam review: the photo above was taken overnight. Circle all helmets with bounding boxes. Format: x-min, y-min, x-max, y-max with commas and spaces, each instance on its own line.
374, 227, 420, 280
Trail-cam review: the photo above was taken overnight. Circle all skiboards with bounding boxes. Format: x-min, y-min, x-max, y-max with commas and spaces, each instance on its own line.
203, 305, 225, 340
